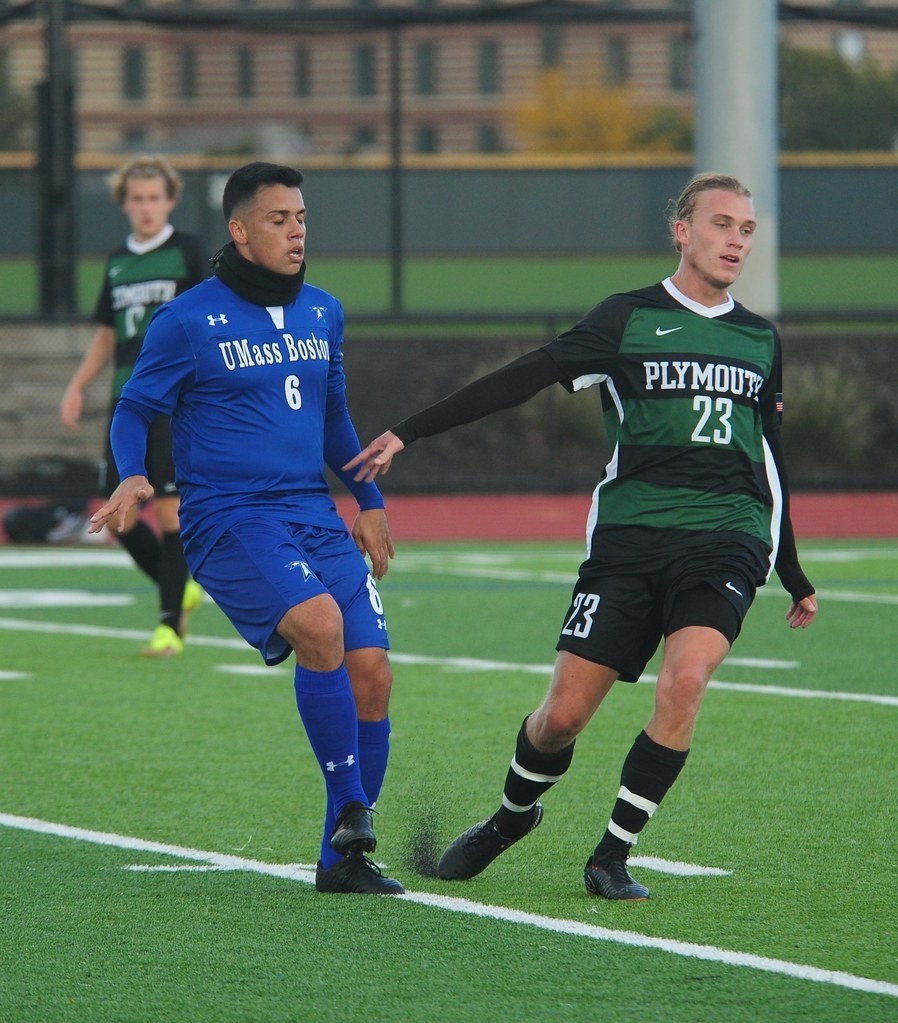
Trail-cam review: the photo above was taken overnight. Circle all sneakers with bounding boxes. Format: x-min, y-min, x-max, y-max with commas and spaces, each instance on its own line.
316, 851, 405, 894
144, 623, 183, 655
438, 802, 544, 881
329, 801, 380, 853
177, 582, 203, 636
582, 848, 650, 901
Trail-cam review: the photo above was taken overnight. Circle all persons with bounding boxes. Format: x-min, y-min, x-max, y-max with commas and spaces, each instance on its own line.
88, 161, 408, 897
61, 159, 202, 655
343, 175, 817, 900
